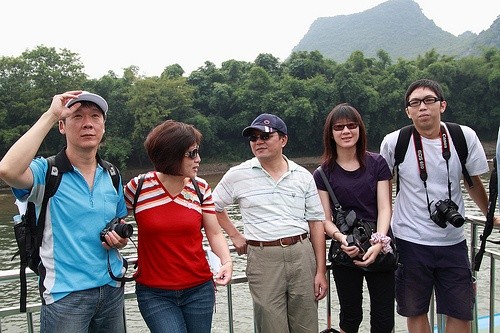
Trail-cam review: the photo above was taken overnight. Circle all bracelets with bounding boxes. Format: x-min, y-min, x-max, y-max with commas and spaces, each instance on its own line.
370, 233, 393, 255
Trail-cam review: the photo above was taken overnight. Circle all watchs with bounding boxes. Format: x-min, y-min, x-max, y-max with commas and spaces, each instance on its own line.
332, 231, 339, 242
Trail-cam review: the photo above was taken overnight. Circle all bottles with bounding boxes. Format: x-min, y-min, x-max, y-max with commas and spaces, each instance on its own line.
13, 215, 22, 225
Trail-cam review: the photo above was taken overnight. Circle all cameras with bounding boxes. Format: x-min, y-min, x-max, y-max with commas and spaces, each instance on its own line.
100, 217, 133, 246
431, 199, 465, 229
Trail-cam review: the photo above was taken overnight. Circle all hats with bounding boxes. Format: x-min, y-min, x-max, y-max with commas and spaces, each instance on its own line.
64, 91, 108, 116
242, 113, 288, 136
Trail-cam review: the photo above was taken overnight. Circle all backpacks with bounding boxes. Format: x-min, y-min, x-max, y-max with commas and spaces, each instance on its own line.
13, 155, 119, 276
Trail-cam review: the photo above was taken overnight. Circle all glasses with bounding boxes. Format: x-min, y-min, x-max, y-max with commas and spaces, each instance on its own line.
247, 131, 275, 141
185, 146, 199, 159
406, 97, 441, 107
332, 122, 359, 131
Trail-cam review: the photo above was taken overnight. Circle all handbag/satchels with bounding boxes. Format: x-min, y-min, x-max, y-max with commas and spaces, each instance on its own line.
328, 210, 398, 272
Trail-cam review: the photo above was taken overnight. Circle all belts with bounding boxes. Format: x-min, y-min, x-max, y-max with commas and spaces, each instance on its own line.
248, 233, 307, 248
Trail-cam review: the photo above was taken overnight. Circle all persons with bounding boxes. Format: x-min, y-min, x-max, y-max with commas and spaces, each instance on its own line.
0, 89, 128, 333
380, 80, 500, 333
212, 114, 330, 333
314, 103, 395, 333
123, 121, 232, 333
